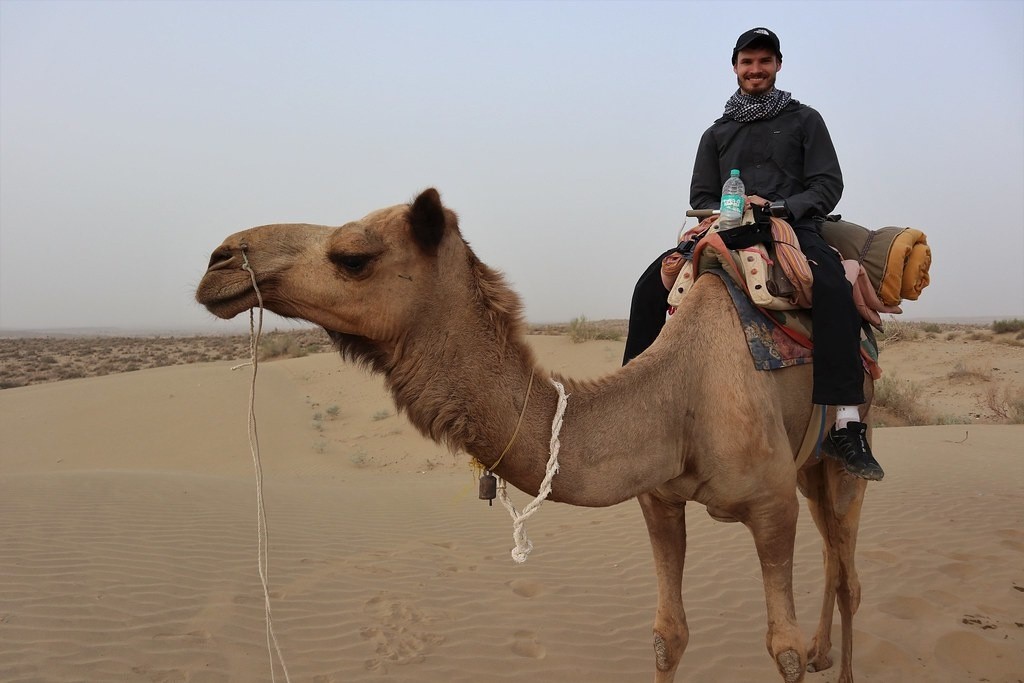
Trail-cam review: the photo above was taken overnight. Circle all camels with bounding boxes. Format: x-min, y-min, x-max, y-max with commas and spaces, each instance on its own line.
195, 187, 878, 683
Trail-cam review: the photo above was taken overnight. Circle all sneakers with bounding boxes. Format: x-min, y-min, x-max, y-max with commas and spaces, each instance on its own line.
820, 421, 884, 481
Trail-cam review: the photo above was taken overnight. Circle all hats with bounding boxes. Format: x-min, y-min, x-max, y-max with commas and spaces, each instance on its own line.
731, 27, 783, 62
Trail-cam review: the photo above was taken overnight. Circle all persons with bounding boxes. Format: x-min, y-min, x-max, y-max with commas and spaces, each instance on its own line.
621, 26, 887, 480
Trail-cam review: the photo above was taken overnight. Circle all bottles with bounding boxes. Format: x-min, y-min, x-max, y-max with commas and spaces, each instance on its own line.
719, 169, 745, 230
